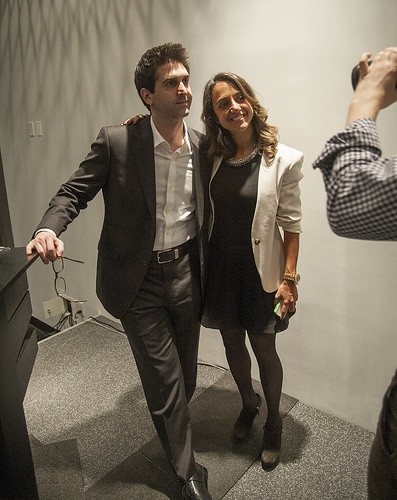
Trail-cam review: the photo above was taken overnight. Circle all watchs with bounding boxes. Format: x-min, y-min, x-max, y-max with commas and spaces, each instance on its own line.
285, 273, 300, 285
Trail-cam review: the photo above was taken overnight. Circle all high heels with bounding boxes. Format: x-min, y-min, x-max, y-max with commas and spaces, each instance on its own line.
261, 416, 282, 468
232, 393, 262, 440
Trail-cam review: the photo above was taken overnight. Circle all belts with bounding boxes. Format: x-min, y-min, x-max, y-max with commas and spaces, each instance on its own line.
152, 238, 196, 263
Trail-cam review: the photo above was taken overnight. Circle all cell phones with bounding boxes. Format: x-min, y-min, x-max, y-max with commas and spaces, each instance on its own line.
274, 299, 288, 319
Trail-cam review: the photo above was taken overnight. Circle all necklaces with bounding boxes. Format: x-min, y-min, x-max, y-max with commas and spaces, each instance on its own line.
226, 148, 258, 167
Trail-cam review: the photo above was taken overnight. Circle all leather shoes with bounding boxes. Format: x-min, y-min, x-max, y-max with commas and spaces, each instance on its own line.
181, 463, 212, 500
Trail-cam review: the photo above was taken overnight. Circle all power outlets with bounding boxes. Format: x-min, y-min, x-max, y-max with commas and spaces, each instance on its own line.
75, 302, 85, 317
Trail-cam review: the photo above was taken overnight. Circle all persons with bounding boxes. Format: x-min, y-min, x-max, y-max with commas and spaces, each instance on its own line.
26, 42, 212, 500
121, 72, 305, 470
312, 47, 397, 500
